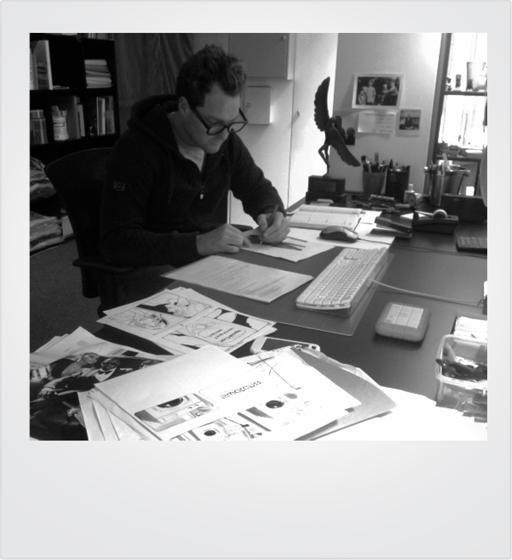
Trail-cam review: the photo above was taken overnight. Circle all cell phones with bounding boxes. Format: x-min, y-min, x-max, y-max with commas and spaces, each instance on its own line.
249, 335, 320, 354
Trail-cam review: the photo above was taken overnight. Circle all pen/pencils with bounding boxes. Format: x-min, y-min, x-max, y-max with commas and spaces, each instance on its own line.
360, 150, 386, 171
259, 204, 279, 248
390, 160, 410, 171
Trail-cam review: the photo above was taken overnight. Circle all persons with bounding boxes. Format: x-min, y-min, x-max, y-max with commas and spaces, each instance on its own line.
97, 43, 290, 301
358, 80, 397, 106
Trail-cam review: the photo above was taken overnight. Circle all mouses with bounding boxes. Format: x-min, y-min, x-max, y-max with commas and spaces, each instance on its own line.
319, 225, 359, 242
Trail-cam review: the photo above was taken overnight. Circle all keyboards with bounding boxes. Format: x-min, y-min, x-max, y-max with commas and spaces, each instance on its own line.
295, 246, 391, 320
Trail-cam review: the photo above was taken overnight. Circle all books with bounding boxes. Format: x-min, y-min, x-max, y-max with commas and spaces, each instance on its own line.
289, 204, 365, 233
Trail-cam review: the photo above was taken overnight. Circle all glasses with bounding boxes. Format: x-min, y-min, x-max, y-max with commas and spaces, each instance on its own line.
181, 92, 248, 137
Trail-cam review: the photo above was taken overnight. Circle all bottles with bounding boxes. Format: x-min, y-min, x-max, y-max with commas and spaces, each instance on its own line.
31, 110, 48, 146
403, 184, 415, 204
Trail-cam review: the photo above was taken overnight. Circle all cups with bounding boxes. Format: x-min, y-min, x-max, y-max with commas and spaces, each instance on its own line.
361, 171, 385, 199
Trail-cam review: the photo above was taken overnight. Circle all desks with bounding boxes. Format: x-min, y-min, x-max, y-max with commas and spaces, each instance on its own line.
29, 178, 488, 440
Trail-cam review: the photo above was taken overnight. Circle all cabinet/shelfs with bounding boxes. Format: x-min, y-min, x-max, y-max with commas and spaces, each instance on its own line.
30, 33, 123, 253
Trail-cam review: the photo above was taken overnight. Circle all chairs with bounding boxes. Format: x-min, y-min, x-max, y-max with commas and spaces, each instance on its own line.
35, 130, 258, 320
